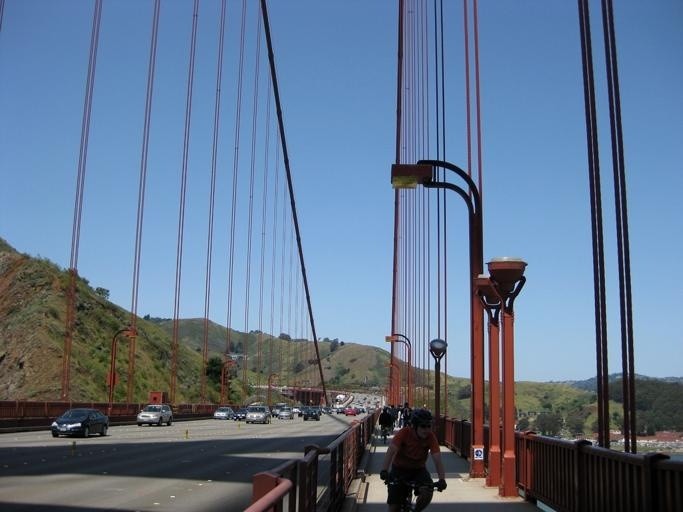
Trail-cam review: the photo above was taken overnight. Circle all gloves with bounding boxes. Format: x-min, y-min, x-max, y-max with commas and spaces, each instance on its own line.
437, 480, 447, 492
380, 470, 388, 480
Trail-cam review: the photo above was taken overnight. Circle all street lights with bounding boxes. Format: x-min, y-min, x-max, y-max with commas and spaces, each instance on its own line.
221, 359, 234, 409
268, 373, 313, 404
388, 157, 484, 475
475, 254, 528, 498
473, 271, 504, 489
104, 327, 137, 418
427, 337, 447, 444
383, 333, 414, 410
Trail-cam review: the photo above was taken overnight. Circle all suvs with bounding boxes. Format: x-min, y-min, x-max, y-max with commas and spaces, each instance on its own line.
136, 404, 173, 427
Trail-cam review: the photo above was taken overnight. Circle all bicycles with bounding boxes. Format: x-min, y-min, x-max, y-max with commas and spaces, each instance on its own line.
379, 473, 444, 512
380, 425, 390, 445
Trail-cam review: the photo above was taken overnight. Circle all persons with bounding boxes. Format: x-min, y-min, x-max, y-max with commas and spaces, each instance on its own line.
379, 402, 447, 512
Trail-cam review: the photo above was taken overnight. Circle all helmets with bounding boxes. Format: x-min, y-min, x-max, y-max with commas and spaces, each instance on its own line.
412, 408, 433, 423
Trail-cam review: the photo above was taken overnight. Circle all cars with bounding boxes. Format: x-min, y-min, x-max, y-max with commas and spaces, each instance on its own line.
234, 408, 247, 421
50, 407, 110, 439
213, 407, 234, 420
336, 392, 380, 417
246, 402, 337, 426
